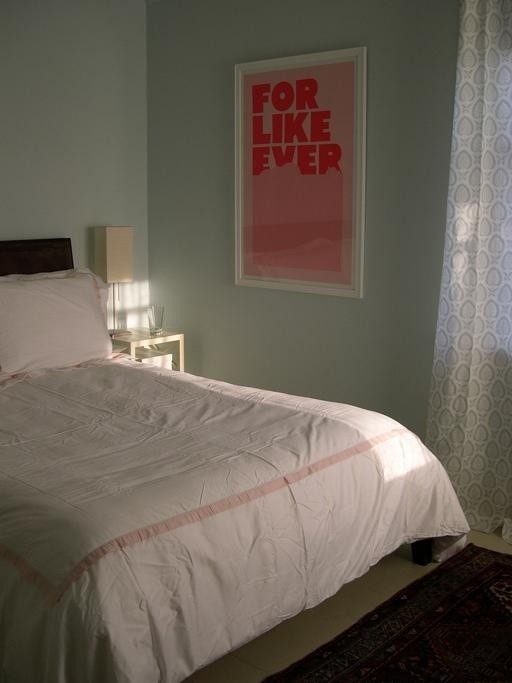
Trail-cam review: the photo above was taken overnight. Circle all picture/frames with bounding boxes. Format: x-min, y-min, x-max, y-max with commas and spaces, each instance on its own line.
232, 43, 371, 300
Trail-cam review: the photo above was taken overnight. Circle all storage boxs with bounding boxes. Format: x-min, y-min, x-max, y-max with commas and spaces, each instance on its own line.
134, 346, 174, 370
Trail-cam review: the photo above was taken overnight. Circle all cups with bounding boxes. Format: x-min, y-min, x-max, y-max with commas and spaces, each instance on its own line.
145, 304, 164, 337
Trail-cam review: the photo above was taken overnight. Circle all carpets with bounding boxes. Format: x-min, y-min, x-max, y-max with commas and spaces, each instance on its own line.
256, 539, 512, 682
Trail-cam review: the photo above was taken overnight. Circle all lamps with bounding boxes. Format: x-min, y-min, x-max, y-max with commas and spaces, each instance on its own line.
92, 224, 136, 337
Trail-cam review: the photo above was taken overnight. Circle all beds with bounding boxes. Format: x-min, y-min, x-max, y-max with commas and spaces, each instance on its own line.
0, 236, 470, 682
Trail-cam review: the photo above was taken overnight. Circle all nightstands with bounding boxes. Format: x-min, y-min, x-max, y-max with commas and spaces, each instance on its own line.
109, 327, 186, 373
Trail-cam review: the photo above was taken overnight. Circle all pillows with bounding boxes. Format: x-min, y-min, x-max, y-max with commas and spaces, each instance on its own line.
0, 263, 114, 379
